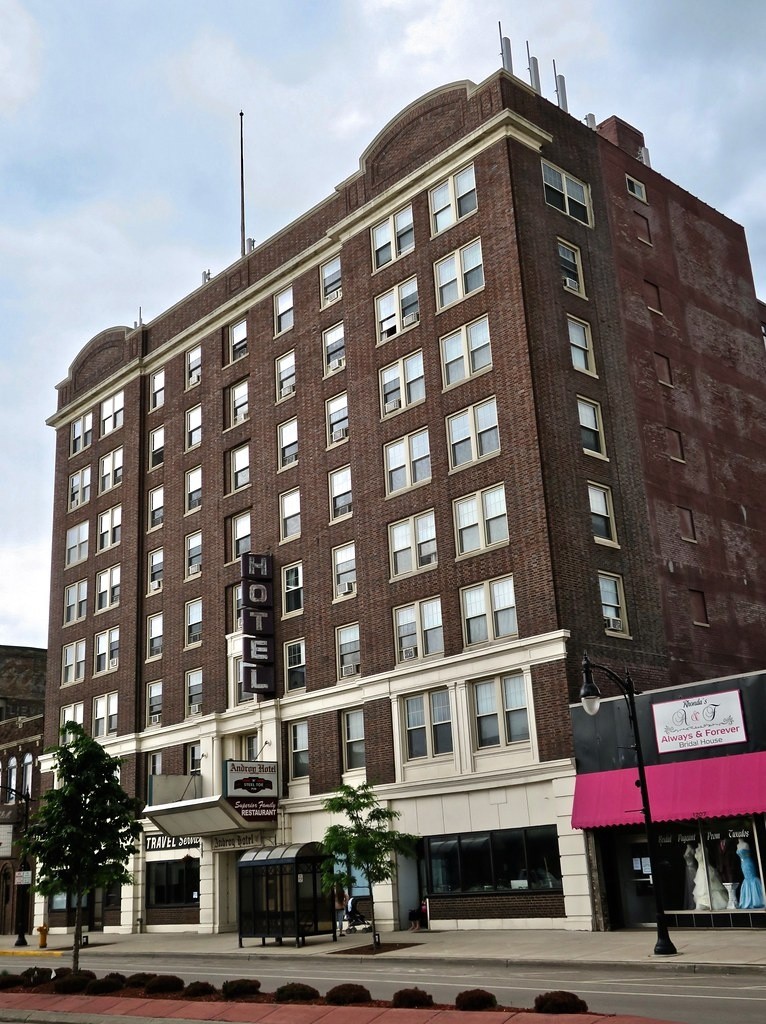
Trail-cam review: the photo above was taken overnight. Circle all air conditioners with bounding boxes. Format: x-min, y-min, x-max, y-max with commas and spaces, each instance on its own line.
190, 375, 199, 387
282, 385, 293, 397
337, 582, 353, 594
109, 658, 118, 669
328, 290, 338, 303
332, 428, 346, 442
604, 617, 623, 631
190, 564, 201, 575
338, 505, 349, 516
330, 360, 341, 372
403, 647, 415, 661
190, 704, 201, 715
343, 664, 357, 677
152, 715, 159, 723
564, 277, 579, 292
150, 580, 161, 591
385, 399, 400, 413
404, 312, 418, 327
236, 413, 247, 423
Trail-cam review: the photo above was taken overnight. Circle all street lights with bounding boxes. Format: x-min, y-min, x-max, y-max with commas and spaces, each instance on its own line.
579, 647, 676, 955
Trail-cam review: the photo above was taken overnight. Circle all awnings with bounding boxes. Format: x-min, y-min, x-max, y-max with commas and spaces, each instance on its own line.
571, 751, 766, 829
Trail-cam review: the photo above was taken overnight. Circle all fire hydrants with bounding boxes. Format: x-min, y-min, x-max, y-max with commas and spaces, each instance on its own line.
36, 922, 49, 948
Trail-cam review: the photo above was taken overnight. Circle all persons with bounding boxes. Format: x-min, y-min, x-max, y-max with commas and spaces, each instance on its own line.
684, 844, 696, 909
693, 844, 729, 910
334, 882, 347, 936
736, 839, 764, 909
408, 897, 427, 932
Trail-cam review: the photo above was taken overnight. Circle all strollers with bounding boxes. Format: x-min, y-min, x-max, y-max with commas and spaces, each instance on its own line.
343, 897, 373, 934
